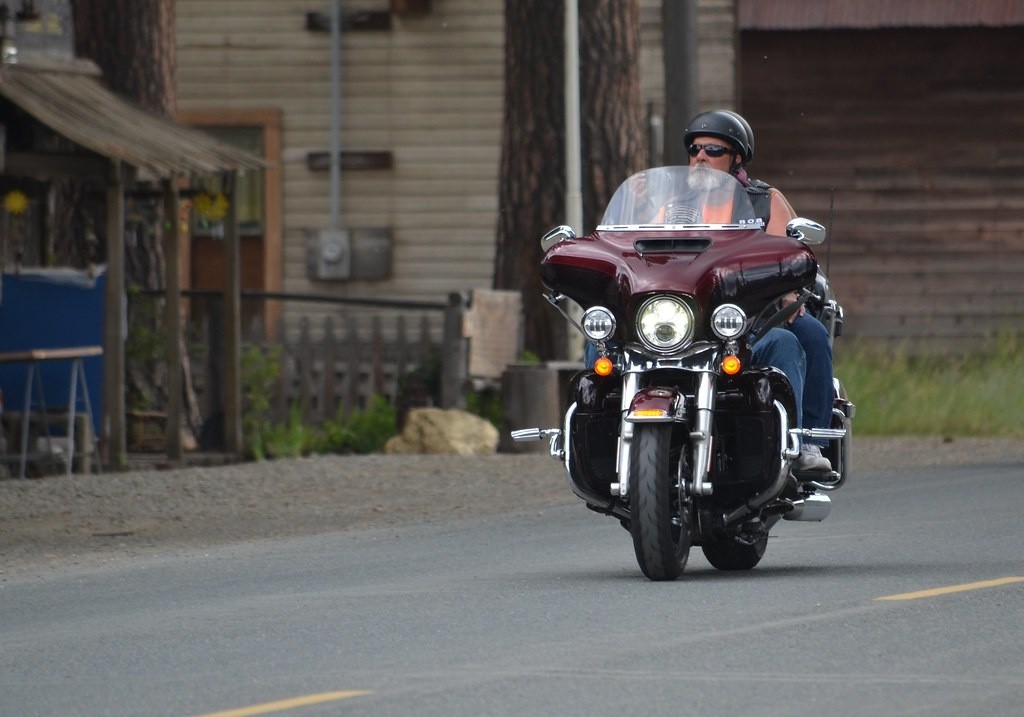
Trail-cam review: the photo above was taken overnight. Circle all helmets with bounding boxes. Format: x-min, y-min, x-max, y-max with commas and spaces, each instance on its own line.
683, 111, 748, 158
718, 109, 756, 162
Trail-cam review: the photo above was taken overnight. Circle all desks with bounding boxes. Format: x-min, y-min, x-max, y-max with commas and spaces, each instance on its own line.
0, 345, 105, 482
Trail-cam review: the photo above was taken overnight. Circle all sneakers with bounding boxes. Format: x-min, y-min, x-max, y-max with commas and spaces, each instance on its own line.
792, 445, 832, 474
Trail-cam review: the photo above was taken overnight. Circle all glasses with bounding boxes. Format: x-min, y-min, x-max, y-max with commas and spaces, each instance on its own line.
687, 144, 736, 159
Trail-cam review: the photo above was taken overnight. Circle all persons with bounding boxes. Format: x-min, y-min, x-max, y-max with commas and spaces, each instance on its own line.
586, 108, 841, 482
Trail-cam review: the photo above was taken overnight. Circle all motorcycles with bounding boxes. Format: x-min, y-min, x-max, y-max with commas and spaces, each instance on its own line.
510, 165, 857, 582
